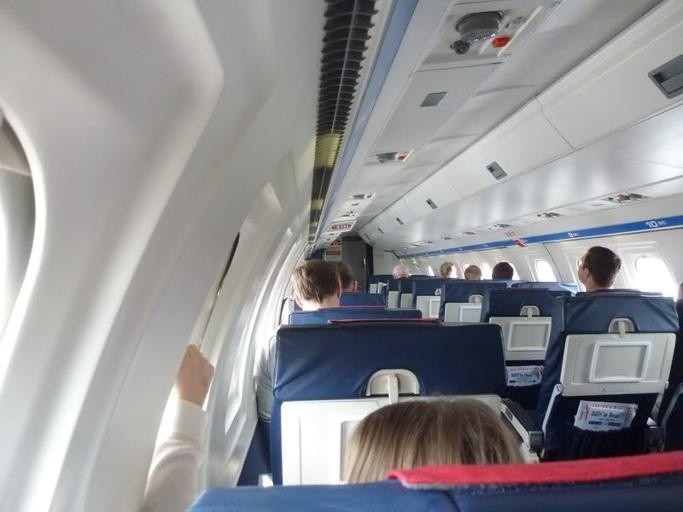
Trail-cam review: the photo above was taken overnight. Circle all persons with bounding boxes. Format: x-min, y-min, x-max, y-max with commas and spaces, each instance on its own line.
251, 257, 344, 437
463, 264, 481, 281
137, 341, 531, 512
575, 245, 623, 292
439, 261, 454, 278
380, 262, 414, 304
491, 261, 514, 281
323, 260, 357, 295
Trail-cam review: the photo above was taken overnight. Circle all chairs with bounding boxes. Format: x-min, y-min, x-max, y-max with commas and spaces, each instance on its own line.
184, 274, 682, 511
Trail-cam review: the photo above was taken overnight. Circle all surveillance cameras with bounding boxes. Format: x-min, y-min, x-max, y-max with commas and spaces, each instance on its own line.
454, 41, 470, 56
